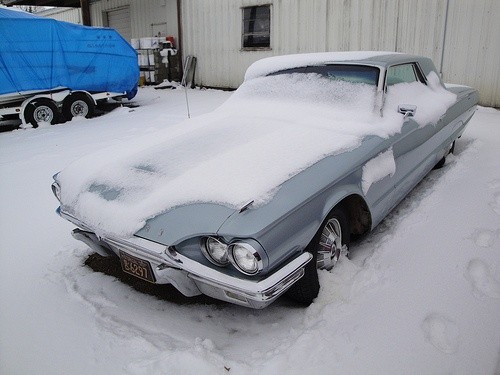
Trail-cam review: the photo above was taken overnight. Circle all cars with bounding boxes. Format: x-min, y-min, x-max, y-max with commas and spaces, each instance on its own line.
52, 52, 480, 309
